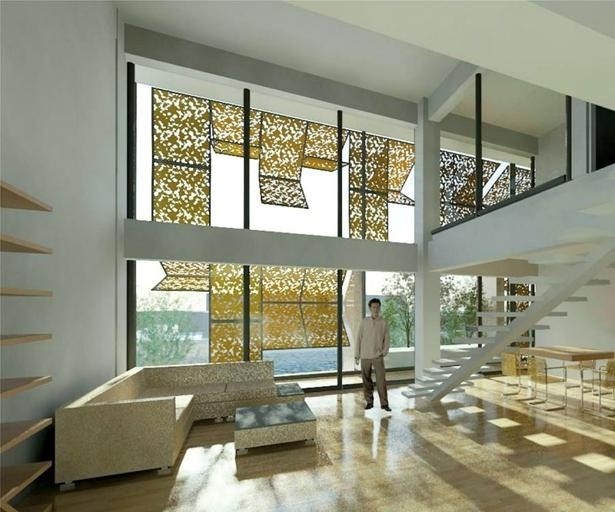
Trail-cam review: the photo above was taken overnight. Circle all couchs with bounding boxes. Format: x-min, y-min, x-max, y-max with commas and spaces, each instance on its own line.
53, 359, 274, 491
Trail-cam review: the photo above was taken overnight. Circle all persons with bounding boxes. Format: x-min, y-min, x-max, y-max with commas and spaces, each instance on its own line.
355, 299, 392, 411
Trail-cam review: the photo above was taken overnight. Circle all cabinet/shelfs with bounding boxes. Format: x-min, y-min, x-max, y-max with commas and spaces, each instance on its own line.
0, 180, 53, 512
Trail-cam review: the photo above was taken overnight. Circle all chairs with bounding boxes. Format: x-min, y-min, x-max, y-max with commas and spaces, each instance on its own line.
501, 346, 615, 417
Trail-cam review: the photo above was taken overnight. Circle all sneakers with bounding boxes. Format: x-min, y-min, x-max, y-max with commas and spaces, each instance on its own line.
381, 405, 392, 411
364, 403, 373, 409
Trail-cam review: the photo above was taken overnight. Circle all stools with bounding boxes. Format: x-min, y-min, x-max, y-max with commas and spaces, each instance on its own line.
276, 383, 303, 400
